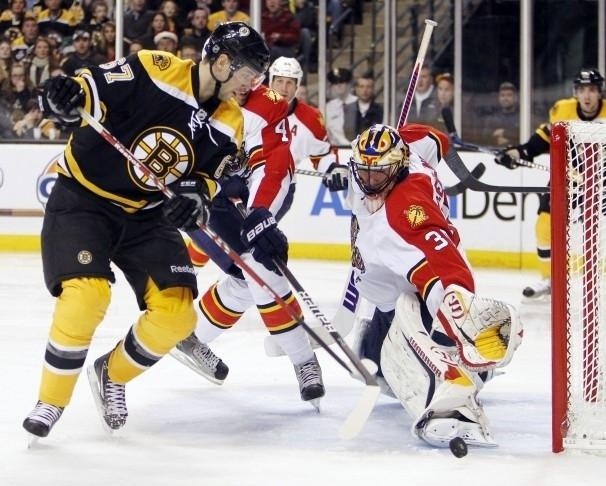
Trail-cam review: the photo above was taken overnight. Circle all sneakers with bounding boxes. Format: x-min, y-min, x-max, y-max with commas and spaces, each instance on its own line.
94, 354, 128, 430
176, 333, 228, 380
294, 353, 324, 401
523, 282, 550, 297
23, 398, 64, 439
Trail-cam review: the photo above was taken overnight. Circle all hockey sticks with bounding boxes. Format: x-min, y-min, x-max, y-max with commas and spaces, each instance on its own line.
230, 196, 379, 443
264, 17, 439, 355
440, 105, 550, 171
446, 146, 552, 193
291, 162, 484, 197
74, 106, 378, 381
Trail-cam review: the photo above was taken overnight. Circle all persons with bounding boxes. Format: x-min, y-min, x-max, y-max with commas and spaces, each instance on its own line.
342, 122, 501, 451
173, 77, 328, 403
325, 62, 520, 149
21, 21, 272, 438
183, 55, 338, 278
2, 0, 359, 140
492, 66, 606, 301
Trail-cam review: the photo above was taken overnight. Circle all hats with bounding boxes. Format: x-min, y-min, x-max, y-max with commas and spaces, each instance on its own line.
328, 68, 352, 83
435, 72, 452, 82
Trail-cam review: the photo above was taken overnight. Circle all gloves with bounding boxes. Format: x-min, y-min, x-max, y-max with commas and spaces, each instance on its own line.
240, 207, 287, 275
321, 165, 348, 191
46, 76, 85, 126
161, 179, 210, 231
495, 146, 533, 169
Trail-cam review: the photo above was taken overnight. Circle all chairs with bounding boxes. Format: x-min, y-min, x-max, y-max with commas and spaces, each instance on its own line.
297, 28, 311, 86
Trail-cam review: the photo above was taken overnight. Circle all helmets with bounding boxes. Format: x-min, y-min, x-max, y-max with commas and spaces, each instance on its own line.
204, 21, 270, 91
268, 56, 303, 86
573, 69, 603, 89
351, 124, 408, 199
